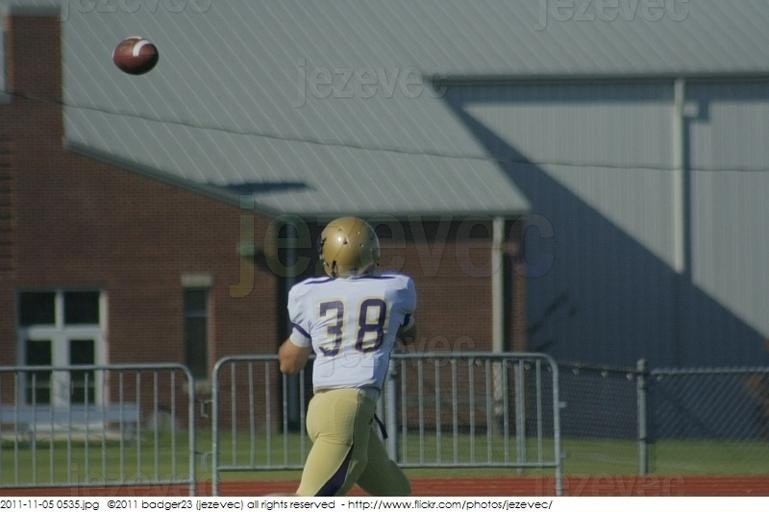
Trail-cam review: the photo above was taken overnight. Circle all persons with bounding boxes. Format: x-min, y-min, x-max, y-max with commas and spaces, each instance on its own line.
276, 216, 418, 495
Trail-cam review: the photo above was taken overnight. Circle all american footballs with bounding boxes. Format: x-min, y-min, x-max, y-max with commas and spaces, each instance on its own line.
112, 36, 157, 76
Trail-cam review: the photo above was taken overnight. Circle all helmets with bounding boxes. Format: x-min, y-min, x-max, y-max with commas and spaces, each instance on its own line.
319, 216, 380, 277
